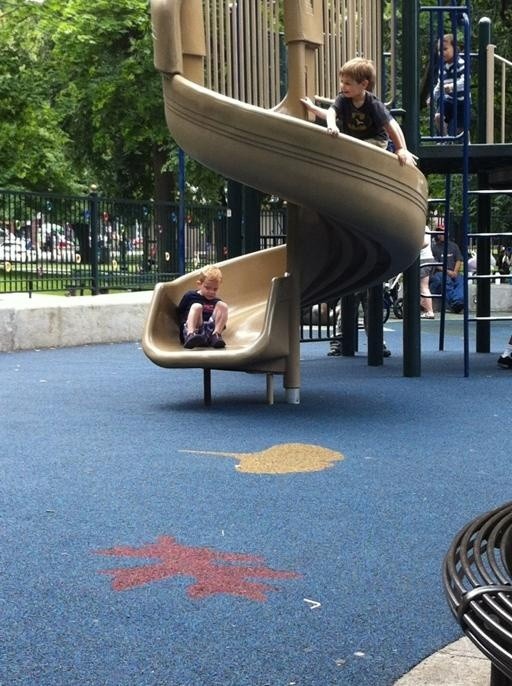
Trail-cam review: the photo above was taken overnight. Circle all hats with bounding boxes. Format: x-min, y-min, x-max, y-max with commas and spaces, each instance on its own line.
436, 224, 445, 231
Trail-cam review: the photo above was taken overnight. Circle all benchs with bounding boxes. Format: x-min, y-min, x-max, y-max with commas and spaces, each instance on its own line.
62, 269, 143, 296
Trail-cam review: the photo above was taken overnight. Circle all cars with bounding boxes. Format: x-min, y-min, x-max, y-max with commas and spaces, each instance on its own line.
0, 221, 155, 262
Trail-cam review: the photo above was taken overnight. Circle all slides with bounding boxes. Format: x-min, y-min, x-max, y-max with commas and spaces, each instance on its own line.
144, 72, 428, 404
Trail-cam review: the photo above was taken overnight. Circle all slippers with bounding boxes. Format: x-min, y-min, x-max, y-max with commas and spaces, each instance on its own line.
420, 311, 434, 319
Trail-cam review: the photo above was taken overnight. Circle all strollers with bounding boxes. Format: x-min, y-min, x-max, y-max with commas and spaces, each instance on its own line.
364, 272, 405, 329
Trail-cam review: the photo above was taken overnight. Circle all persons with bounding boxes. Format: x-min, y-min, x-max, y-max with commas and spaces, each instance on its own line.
420, 224, 512, 318
299, 81, 419, 167
327, 282, 392, 357
426, 33, 473, 145
497, 335, 512, 369
326, 56, 406, 167
176, 267, 230, 350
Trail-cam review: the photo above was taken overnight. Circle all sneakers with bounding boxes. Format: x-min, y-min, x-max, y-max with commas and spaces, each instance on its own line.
184, 332, 204, 349
384, 344, 391, 357
497, 355, 511, 368
208, 331, 225, 348
328, 336, 343, 355
